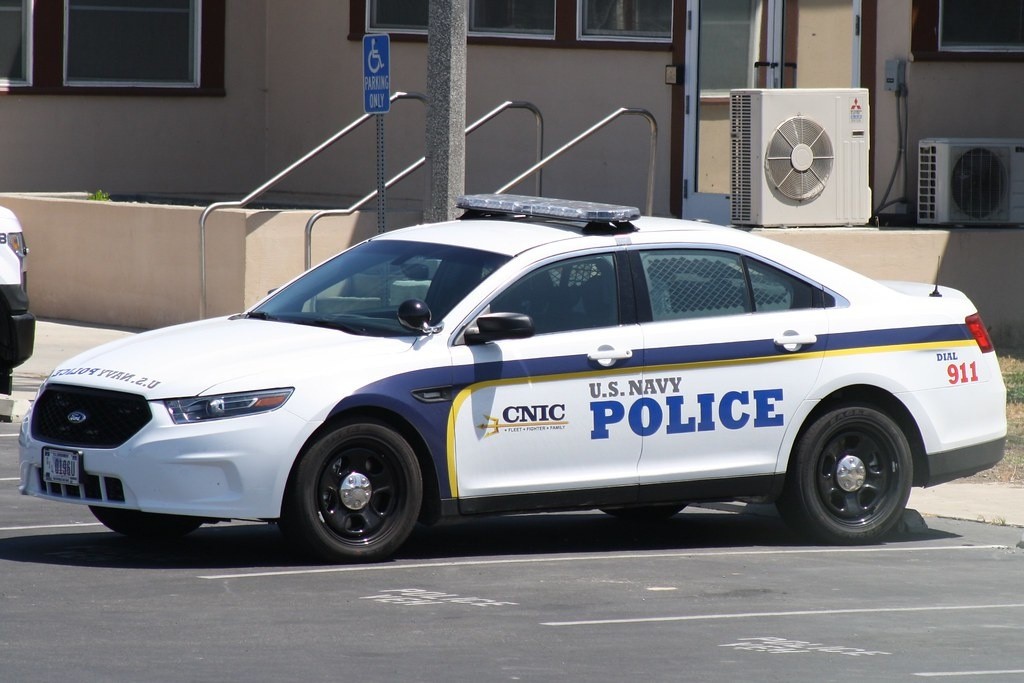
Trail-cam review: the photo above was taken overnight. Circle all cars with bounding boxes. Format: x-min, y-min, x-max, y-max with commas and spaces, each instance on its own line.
12, 191, 1006, 565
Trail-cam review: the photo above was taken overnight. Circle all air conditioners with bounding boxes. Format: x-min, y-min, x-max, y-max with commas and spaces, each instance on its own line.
914, 136, 1024, 228
727, 85, 874, 229
